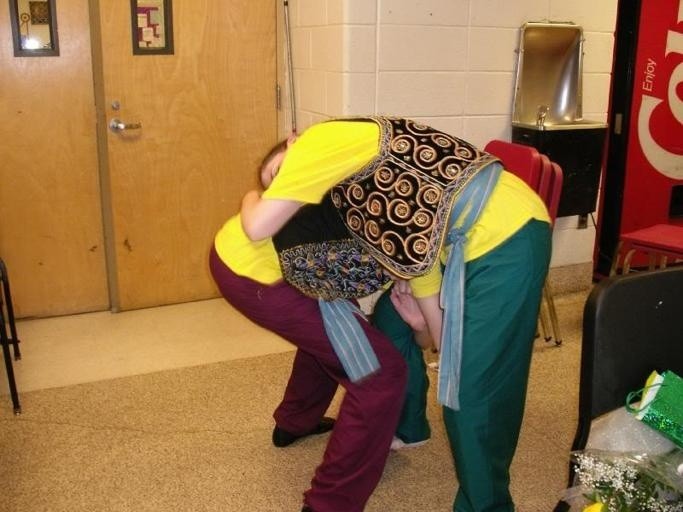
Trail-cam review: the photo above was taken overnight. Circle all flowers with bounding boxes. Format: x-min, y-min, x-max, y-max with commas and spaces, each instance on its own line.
567, 445, 679, 512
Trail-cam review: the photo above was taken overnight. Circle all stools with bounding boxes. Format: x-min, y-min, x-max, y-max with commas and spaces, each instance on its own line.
611, 220, 682, 278
0, 257, 22, 415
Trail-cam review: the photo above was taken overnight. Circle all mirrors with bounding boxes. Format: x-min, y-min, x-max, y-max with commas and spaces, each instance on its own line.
510, 20, 584, 123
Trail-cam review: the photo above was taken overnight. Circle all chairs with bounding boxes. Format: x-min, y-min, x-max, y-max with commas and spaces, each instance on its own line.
482, 140, 563, 348
552, 262, 682, 512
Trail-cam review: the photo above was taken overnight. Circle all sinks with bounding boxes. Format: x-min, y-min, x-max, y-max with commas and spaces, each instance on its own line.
513, 118, 609, 132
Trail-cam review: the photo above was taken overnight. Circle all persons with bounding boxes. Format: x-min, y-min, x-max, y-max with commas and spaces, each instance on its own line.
209, 197, 445, 511
241, 114, 552, 511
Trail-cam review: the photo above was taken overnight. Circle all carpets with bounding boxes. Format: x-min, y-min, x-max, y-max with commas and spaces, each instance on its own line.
0, 293, 595, 511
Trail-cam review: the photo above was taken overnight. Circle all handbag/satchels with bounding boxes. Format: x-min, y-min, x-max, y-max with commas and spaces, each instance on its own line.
626, 370, 683, 449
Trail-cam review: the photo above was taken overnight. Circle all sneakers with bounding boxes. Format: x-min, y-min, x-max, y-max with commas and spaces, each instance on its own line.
272, 416, 335, 447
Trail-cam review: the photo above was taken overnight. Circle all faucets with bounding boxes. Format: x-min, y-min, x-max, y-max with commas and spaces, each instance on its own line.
535, 104, 550, 126
